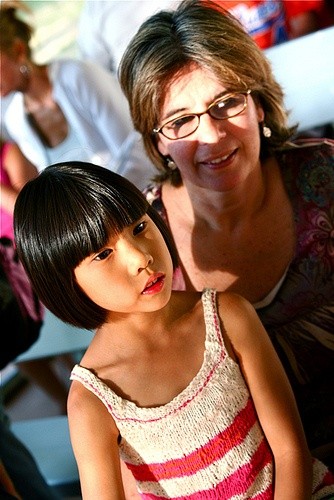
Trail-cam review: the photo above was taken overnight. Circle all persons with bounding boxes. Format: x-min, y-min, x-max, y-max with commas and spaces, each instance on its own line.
77, 0, 334, 74
116, 0, 334, 476
14, 162, 334, 500
0, 6, 156, 325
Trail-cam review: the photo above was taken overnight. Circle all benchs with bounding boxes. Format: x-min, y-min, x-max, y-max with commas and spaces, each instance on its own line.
10, 415, 83, 498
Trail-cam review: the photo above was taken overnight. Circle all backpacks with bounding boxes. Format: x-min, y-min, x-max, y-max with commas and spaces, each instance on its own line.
0, 236, 44, 373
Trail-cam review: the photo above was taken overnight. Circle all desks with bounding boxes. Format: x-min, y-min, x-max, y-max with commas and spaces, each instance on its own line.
14, 308, 97, 416
262, 25, 334, 134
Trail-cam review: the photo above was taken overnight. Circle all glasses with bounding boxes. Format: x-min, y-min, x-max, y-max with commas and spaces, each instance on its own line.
153, 89, 252, 140
